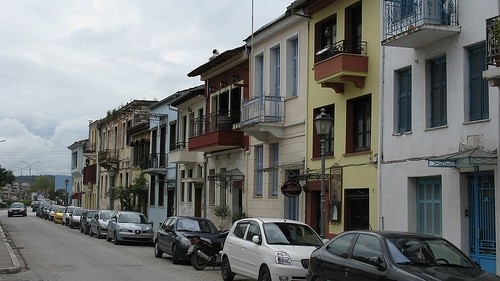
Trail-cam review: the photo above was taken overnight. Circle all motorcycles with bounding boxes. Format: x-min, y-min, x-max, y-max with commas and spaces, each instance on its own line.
187, 229, 229, 270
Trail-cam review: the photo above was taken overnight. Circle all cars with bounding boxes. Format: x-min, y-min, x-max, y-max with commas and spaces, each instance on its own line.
8, 203, 27, 217
221, 218, 330, 281
107, 211, 154, 245
306, 230, 500, 281
31, 199, 117, 239
154, 216, 220, 265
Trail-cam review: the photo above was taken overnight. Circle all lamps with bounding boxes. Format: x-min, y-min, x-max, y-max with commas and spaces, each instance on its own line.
209, 86, 215, 93
219, 80, 225, 88
285, 9, 311, 19
232, 76, 249, 88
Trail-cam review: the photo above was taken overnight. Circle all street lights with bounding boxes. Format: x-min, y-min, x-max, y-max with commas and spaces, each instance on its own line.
21, 160, 40, 199
314, 107, 334, 240
64, 178, 69, 206
13, 165, 29, 201
